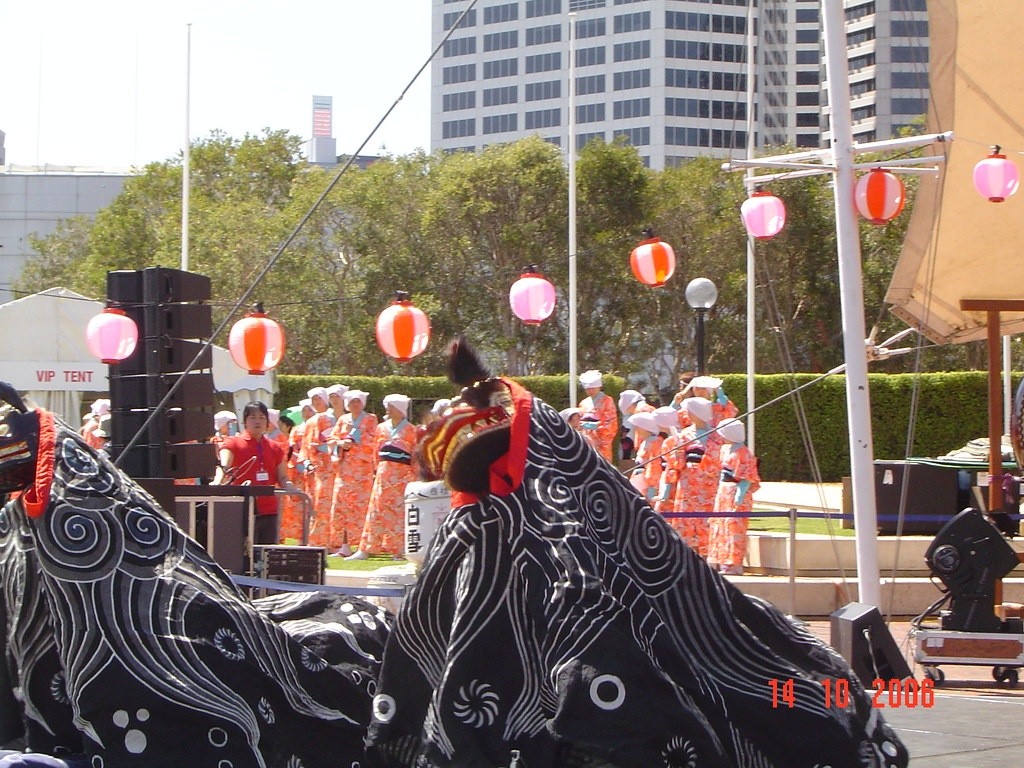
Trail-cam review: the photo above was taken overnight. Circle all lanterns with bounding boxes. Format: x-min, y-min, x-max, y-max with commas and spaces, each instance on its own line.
629, 238, 676, 288
376, 300, 432, 362
508, 273, 557, 325
229, 312, 285, 376
972, 155, 1020, 203
86, 308, 138, 365
853, 169, 906, 225
739, 192, 786, 241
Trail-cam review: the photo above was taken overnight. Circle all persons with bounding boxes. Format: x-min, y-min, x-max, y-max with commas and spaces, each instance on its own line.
209, 410, 241, 438
262, 384, 421, 561
210, 401, 302, 545
559, 370, 760, 576
77, 398, 111, 449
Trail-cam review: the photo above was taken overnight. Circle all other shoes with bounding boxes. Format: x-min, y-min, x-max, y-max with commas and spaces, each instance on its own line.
717, 563, 743, 575
338, 544, 352, 556
327, 548, 336, 554
344, 550, 368, 561
393, 556, 404, 560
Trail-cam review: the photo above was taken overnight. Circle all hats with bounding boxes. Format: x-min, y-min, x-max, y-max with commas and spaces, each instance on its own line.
325, 384, 349, 399
382, 394, 410, 417
83, 398, 110, 420
693, 387, 713, 395
343, 390, 370, 411
716, 418, 745, 443
306, 386, 329, 408
618, 390, 646, 413
627, 412, 659, 436
92, 414, 111, 438
680, 397, 712, 423
579, 369, 602, 389
645, 405, 678, 428
558, 407, 581, 421
267, 408, 280, 429
214, 410, 237, 430
430, 398, 451, 415
299, 399, 317, 413
